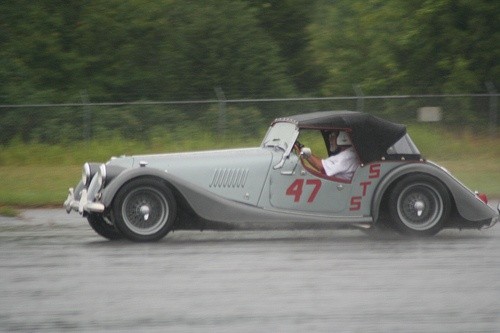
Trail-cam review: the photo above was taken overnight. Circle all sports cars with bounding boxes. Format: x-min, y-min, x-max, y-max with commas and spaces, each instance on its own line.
62, 111, 500, 243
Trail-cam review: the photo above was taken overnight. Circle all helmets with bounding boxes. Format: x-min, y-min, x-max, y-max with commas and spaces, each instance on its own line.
325, 129, 351, 145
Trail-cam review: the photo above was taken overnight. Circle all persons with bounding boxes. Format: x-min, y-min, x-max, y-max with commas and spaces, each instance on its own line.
294, 128, 362, 183
